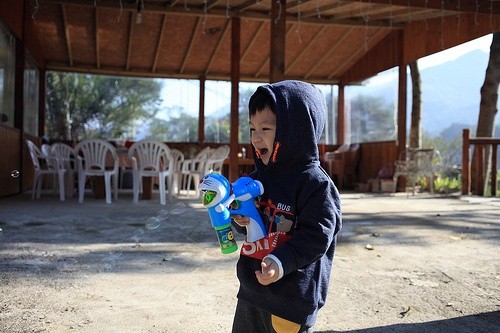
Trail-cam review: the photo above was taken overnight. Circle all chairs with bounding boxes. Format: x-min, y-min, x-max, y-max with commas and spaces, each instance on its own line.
50, 141, 82, 197
325, 143, 361, 191
24, 139, 70, 201
391, 147, 436, 196
41, 143, 75, 196
160, 144, 231, 200
129, 141, 175, 206
74, 138, 121, 204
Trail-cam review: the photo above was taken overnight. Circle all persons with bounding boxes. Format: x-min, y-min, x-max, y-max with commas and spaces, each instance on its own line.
231, 80, 343, 333
